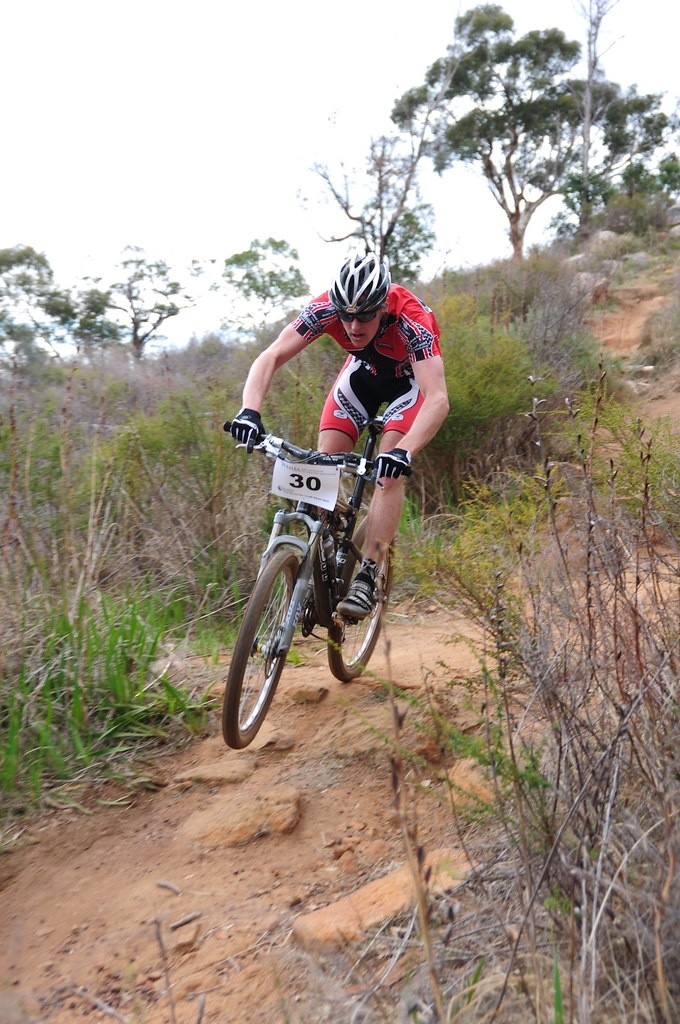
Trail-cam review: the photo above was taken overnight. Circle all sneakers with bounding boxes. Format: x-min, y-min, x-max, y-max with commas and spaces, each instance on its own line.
337, 571, 378, 618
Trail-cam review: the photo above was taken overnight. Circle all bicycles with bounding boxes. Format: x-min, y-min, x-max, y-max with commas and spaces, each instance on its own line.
222, 420, 412, 751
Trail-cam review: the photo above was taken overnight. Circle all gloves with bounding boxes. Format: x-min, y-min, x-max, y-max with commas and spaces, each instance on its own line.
375, 448, 411, 480
229, 408, 265, 454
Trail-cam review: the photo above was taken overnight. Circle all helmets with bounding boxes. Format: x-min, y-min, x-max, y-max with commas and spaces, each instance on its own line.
328, 258, 391, 314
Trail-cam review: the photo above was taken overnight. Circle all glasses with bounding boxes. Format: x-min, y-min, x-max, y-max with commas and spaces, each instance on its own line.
338, 311, 378, 322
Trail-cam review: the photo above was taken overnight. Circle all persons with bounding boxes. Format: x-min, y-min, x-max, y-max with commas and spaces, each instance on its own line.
228, 251, 449, 618
